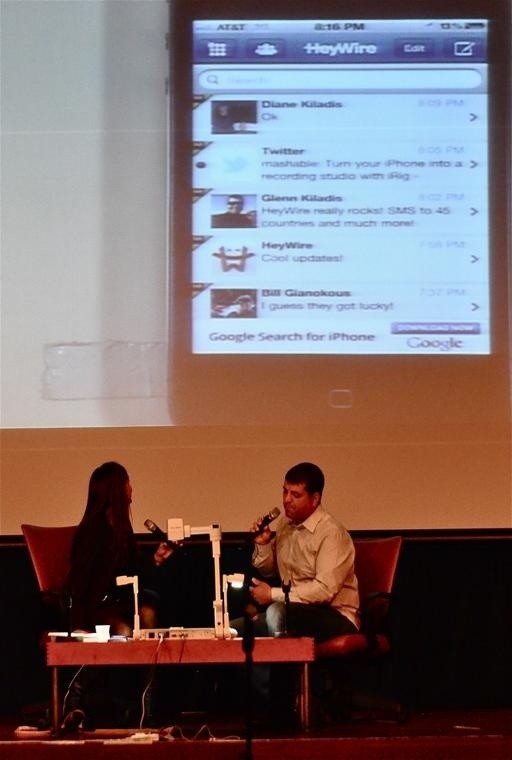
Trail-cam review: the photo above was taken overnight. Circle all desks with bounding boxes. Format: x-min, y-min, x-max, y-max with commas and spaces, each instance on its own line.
45, 637, 314, 733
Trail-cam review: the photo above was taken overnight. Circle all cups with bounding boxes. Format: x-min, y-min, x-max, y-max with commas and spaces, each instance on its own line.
94, 624, 111, 643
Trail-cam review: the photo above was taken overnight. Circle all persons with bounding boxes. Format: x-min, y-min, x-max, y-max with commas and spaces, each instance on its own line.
211, 462, 362, 726
59, 460, 184, 727
213, 195, 255, 228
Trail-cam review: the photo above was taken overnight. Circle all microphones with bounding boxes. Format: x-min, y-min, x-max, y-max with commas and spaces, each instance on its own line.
252, 507, 281, 531
144, 516, 185, 555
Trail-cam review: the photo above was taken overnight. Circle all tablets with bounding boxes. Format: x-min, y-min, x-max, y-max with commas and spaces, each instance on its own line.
164, 1, 511, 432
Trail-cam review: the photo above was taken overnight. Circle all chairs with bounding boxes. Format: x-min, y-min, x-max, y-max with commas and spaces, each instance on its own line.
315, 537, 410, 729
22, 523, 89, 625
